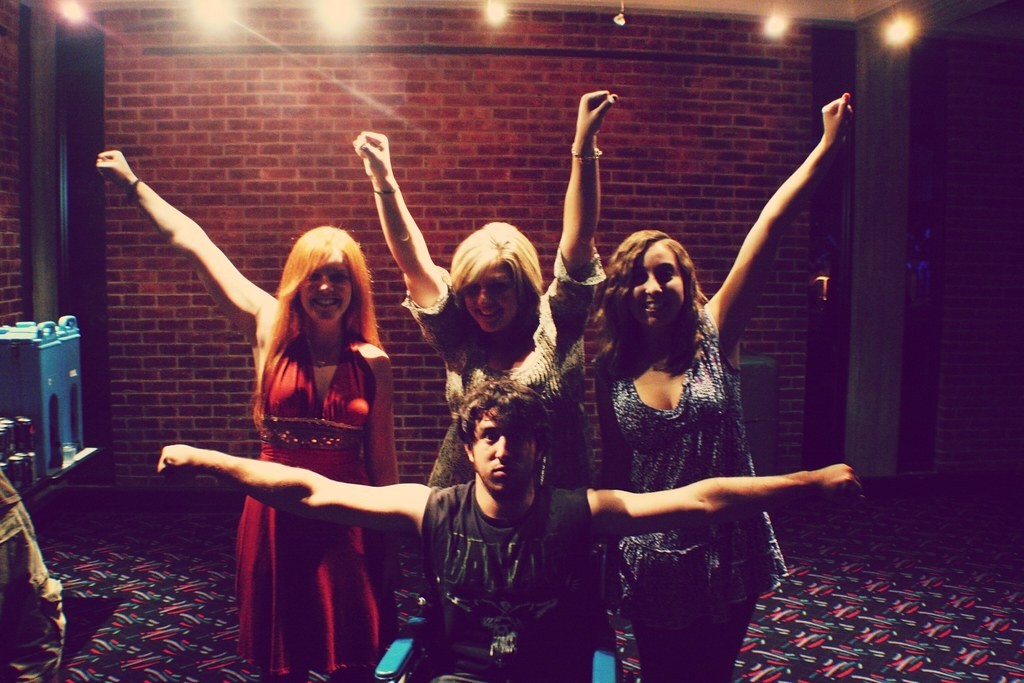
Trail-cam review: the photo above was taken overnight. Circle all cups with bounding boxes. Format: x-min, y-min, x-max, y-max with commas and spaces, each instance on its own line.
61, 442, 78, 468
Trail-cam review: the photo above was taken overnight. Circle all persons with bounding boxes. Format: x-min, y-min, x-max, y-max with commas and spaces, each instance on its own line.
0, 470, 66, 683
97, 150, 399, 683
590, 92, 853, 683
157, 381, 866, 683
356, 92, 618, 489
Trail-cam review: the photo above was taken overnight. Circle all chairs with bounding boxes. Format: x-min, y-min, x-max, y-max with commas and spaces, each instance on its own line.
374, 592, 619, 683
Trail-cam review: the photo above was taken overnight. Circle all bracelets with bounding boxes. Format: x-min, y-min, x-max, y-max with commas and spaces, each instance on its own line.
571, 144, 603, 165
374, 185, 400, 194
131, 179, 141, 189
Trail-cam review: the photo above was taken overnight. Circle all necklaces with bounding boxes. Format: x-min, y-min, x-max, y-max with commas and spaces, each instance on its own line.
316, 360, 331, 368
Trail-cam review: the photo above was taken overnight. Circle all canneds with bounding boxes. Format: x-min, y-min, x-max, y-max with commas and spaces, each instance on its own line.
0, 417, 36, 494
